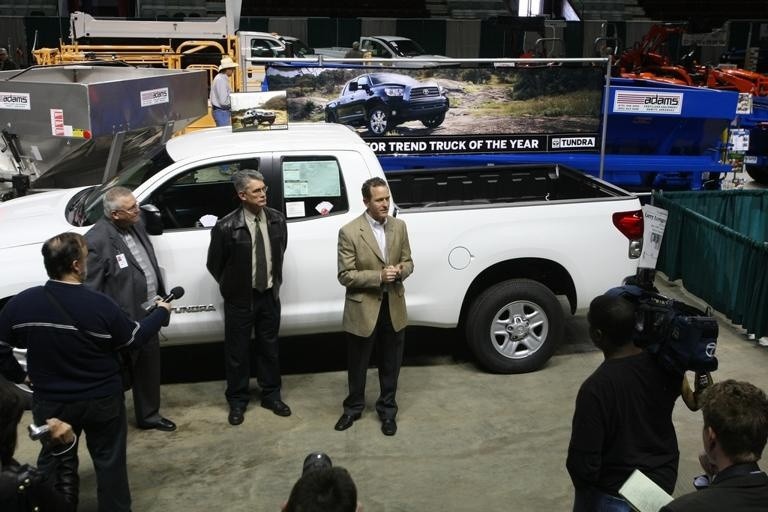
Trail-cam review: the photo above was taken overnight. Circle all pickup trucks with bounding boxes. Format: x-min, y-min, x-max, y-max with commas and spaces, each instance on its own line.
324, 73, 450, 136
241, 109, 277, 128
237, 11, 462, 90
1, 123, 644, 411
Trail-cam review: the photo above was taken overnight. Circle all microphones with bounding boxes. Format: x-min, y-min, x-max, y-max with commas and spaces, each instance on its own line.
162, 287, 184, 303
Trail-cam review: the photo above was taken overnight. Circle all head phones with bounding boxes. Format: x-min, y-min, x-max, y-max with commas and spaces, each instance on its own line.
590, 287, 641, 343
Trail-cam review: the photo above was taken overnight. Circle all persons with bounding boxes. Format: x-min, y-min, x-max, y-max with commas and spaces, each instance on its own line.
0, 377, 79, 512
602, 46, 613, 63
565, 294, 713, 512
0, 232, 171, 512
0, 47, 18, 71
281, 465, 357, 511
658, 377, 768, 512
209, 58, 239, 126
343, 41, 365, 65
334, 176, 414, 435
207, 169, 291, 426
83, 187, 176, 431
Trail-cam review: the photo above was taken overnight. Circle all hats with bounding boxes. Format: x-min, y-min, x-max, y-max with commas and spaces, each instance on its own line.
218, 58, 238, 71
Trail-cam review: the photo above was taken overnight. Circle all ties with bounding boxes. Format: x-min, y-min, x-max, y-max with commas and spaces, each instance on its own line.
255, 217, 267, 293
124, 230, 157, 300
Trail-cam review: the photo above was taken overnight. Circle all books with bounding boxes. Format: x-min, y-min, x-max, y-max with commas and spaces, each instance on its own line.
618, 469, 674, 512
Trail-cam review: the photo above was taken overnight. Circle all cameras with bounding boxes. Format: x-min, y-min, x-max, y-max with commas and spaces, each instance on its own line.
28, 423, 50, 440
303, 452, 333, 480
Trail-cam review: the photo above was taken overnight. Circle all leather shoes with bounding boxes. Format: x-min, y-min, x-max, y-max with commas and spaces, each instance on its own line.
335, 413, 361, 430
382, 418, 396, 435
138, 418, 176, 431
261, 400, 291, 416
229, 406, 247, 424
